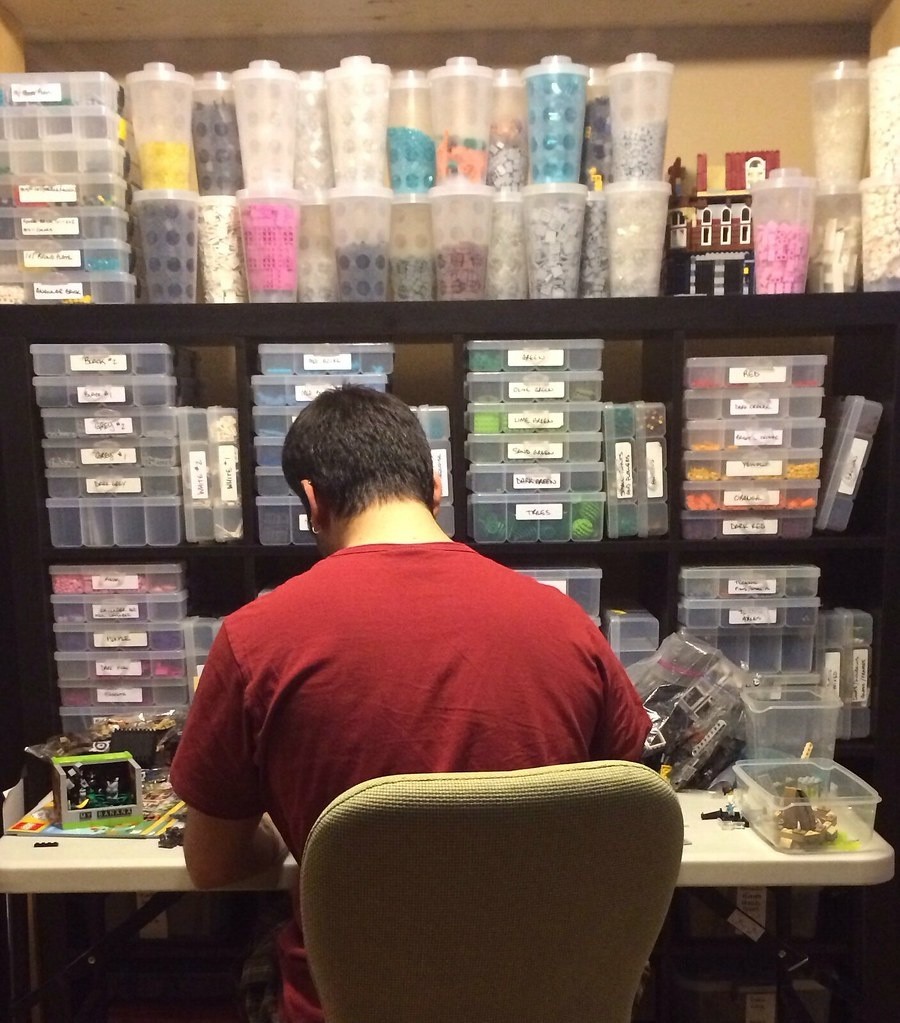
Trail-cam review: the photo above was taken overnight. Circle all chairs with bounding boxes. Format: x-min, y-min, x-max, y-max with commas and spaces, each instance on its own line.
299, 761, 684, 1023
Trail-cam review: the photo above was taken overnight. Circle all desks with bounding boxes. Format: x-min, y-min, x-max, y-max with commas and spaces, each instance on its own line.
0, 746, 895, 1023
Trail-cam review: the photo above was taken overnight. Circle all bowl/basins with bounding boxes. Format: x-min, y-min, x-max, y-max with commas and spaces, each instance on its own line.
729, 755, 882, 855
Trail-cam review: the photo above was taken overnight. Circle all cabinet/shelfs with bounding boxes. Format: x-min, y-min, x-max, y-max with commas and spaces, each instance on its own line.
0, 291, 900, 1023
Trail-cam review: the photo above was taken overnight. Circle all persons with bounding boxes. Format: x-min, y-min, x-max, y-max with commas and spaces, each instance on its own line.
169, 377, 653, 1023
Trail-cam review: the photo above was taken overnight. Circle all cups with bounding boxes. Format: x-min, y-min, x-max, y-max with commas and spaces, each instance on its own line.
749, 167, 818, 293
121, 50, 678, 303
857, 44, 900, 295
804, 175, 863, 293
807, 59, 869, 190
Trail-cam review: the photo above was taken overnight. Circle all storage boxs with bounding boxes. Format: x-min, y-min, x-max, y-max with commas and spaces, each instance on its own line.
104, 891, 236, 941
673, 886, 849, 941
669, 975, 832, 1023
